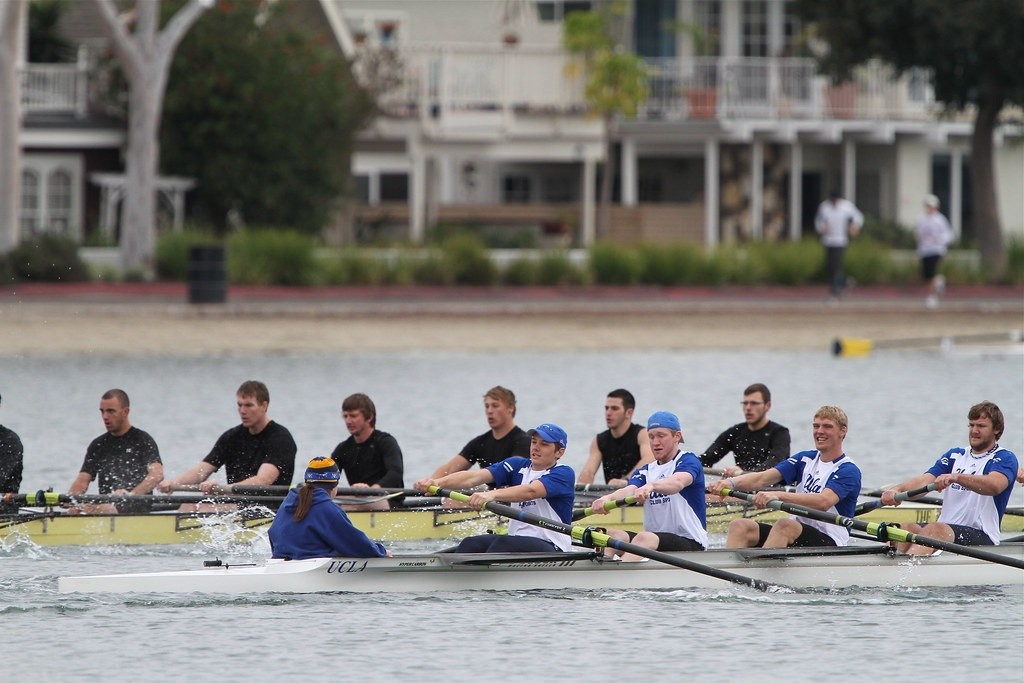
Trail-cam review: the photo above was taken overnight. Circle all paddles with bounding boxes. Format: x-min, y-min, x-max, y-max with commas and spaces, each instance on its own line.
429, 483, 773, 594
496, 494, 646, 535
574, 484, 796, 494
721, 488, 1024, 569
404, 499, 594, 507
855, 483, 937, 516
0, 491, 403, 505
170, 484, 475, 496
703, 466, 1024, 516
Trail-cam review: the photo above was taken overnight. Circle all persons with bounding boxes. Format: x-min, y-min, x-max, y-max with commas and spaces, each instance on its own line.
709, 406, 861, 548
592, 410, 708, 562
420, 423, 575, 553
267, 456, 392, 561
61, 389, 164, 515
577, 389, 655, 486
813, 187, 865, 302
330, 393, 405, 510
411, 386, 531, 508
912, 195, 955, 310
156, 380, 296, 513
0, 394, 24, 514
880, 400, 1019, 554
697, 383, 790, 501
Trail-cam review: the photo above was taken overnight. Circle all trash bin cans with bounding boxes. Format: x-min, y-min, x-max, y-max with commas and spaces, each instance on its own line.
185, 244, 225, 304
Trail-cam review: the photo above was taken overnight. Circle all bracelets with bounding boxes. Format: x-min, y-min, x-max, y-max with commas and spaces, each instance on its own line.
726, 478, 736, 489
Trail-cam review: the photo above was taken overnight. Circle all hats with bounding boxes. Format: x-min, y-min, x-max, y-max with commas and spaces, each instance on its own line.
304, 457, 340, 485
526, 423, 566, 447
647, 411, 684, 443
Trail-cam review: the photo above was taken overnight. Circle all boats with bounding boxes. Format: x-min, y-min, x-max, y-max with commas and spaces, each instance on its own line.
1, 489, 1024, 545
57, 542, 1024, 588
